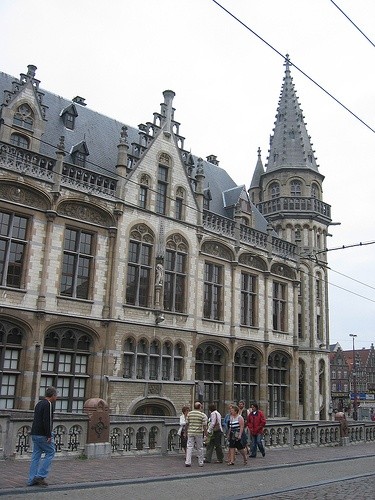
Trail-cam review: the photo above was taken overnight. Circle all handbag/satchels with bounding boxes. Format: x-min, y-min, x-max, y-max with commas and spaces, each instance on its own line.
232, 431, 238, 440
213, 412, 220, 432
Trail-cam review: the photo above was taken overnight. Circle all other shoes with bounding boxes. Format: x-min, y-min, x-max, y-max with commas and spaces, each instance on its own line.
262, 452, 265, 456
249, 455, 256, 458
204, 460, 210, 463
215, 460, 222, 464
185, 464, 191, 467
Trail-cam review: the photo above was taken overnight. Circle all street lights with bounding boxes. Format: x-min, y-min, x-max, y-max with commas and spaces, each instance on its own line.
350, 333, 358, 421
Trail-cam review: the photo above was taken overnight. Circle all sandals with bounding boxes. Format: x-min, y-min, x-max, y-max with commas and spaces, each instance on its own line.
244, 457, 247, 464
226, 462, 234, 465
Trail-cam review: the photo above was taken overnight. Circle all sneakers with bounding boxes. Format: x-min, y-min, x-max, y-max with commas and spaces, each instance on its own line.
30, 482, 39, 486
35, 477, 48, 485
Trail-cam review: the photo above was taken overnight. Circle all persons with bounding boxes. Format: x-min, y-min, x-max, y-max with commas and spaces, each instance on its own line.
177, 407, 189, 453
27, 386, 57, 487
224, 400, 250, 462
248, 403, 266, 457
226, 406, 248, 466
204, 404, 224, 464
185, 402, 207, 467
